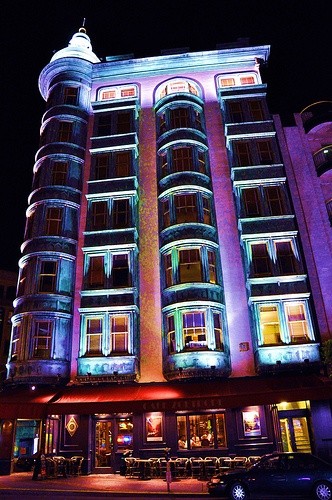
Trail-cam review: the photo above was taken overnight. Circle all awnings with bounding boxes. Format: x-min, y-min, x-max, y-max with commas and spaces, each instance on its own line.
0, 372, 332, 421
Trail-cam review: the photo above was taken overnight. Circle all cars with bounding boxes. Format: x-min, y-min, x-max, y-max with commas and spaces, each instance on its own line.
206, 452, 332, 500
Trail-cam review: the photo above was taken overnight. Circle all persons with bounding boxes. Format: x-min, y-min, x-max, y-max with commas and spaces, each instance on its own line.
185, 434, 210, 449
204, 430, 211, 441
191, 433, 196, 441
210, 432, 214, 443
178, 434, 185, 448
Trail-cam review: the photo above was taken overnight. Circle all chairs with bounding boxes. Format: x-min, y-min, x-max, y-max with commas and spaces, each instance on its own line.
125, 456, 260, 480
45, 456, 84, 479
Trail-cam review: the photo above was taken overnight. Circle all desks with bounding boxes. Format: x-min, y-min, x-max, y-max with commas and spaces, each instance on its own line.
135, 460, 152, 479
193, 460, 212, 480
59, 459, 76, 478
162, 461, 181, 482
224, 459, 243, 468
